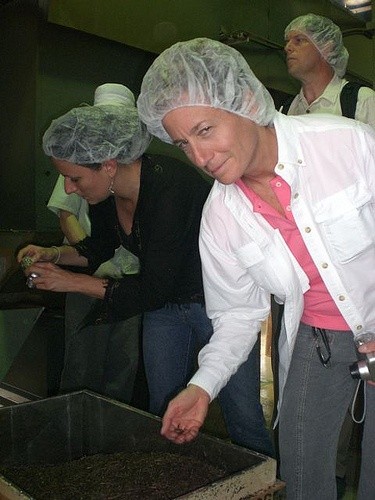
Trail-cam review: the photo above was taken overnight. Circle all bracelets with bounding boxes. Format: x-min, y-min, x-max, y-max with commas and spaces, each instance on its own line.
52, 246, 60, 264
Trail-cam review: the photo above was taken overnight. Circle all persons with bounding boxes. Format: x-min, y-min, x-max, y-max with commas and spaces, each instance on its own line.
137, 39, 375, 500
18, 104, 273, 457
279, 13, 375, 500
46, 84, 143, 405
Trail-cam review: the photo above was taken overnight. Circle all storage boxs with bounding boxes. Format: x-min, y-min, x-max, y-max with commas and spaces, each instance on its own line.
0, 390, 276, 500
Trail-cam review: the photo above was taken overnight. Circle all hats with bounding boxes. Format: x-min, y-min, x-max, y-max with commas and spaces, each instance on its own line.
94, 83, 135, 108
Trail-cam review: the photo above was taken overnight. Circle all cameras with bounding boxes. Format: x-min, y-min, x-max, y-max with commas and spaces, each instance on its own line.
22, 257, 39, 288
350, 338, 375, 383
